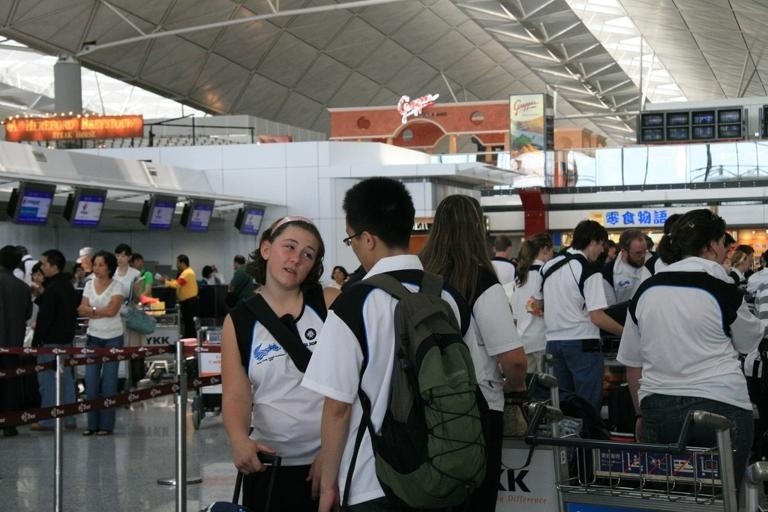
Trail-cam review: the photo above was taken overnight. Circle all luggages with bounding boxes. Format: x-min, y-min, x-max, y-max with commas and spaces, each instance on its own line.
200, 453, 281, 512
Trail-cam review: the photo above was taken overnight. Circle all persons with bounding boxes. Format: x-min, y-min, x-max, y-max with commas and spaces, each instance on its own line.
328, 266, 348, 290
489, 235, 519, 284
299, 177, 472, 512
220, 215, 342, 512
600, 208, 768, 500
510, 232, 553, 373
535, 219, 624, 487
417, 194, 528, 512
0, 241, 254, 435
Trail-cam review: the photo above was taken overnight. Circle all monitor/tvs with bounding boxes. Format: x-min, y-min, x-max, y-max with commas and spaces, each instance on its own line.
640, 108, 742, 142
8, 181, 57, 225
64, 187, 108, 227
235, 204, 266, 235
181, 197, 216, 232
140, 192, 179, 232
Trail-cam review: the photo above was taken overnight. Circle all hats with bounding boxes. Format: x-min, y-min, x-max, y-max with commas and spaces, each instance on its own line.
669, 209, 726, 242
76, 247, 95, 263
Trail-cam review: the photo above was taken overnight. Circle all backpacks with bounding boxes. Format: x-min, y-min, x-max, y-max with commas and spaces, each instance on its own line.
351, 273, 488, 511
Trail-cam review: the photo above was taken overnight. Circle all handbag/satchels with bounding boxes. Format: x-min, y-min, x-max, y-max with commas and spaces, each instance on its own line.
559, 393, 609, 485
121, 305, 155, 334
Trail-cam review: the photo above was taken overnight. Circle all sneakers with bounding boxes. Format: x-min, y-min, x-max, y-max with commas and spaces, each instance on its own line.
0, 422, 113, 436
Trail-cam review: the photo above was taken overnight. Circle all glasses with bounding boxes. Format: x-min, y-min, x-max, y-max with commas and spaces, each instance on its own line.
343, 232, 358, 246
